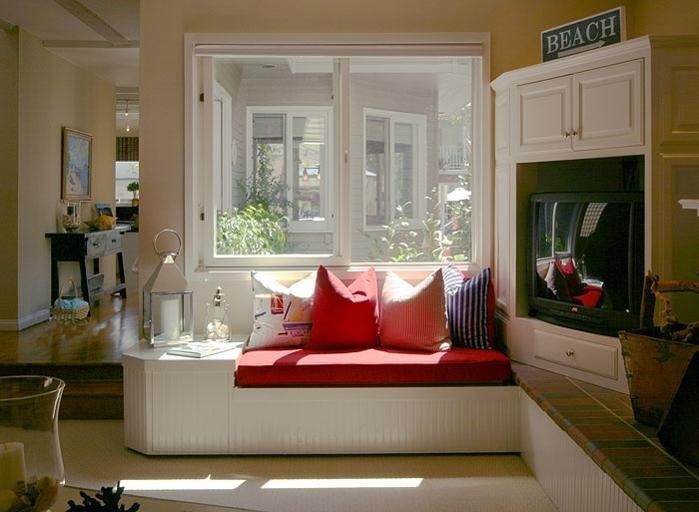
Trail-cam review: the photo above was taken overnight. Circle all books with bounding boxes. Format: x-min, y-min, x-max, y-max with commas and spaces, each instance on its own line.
166, 341, 242, 357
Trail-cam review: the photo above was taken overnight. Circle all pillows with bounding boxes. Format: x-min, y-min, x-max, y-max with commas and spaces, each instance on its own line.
304, 265, 380, 351
443, 263, 496, 350
380, 268, 453, 352
243, 270, 317, 353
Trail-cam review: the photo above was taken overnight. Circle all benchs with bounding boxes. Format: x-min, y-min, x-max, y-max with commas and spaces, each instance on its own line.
121, 340, 520, 454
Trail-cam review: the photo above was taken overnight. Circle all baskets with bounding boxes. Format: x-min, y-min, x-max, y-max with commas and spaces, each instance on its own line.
49, 278, 90, 320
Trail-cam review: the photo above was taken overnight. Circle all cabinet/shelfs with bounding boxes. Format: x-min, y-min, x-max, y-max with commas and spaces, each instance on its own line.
490, 34, 698, 396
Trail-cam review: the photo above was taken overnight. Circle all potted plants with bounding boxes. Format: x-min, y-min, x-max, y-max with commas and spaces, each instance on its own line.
128, 182, 139, 207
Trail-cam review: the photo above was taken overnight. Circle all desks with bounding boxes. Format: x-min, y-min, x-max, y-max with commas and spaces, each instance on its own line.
45, 224, 131, 316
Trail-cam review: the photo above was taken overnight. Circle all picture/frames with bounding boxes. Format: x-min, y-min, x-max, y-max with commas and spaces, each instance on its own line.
62, 126, 93, 202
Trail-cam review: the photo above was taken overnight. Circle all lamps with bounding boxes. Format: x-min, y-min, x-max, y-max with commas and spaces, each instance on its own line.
204, 286, 233, 343
125, 102, 129, 117
142, 229, 193, 349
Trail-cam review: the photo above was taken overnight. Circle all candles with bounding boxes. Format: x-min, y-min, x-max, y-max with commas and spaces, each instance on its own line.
0, 441, 28, 495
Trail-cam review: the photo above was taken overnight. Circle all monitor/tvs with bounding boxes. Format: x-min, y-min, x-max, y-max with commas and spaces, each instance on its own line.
527, 190, 644, 338
